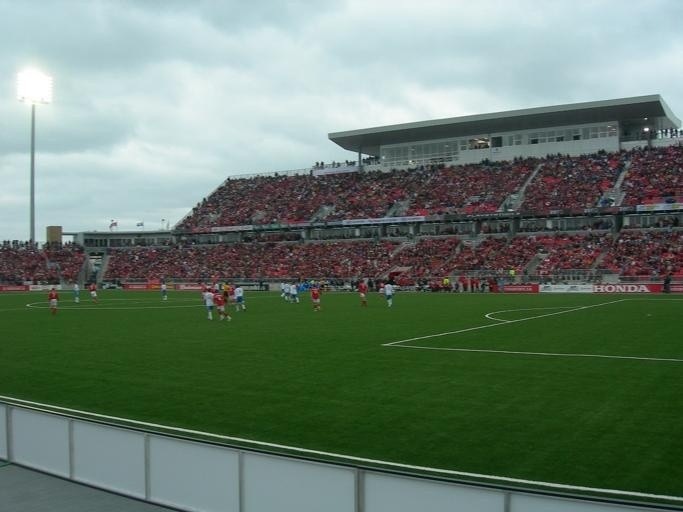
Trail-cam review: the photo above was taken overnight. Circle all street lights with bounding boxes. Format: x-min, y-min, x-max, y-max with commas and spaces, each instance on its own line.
16, 66, 53, 244
